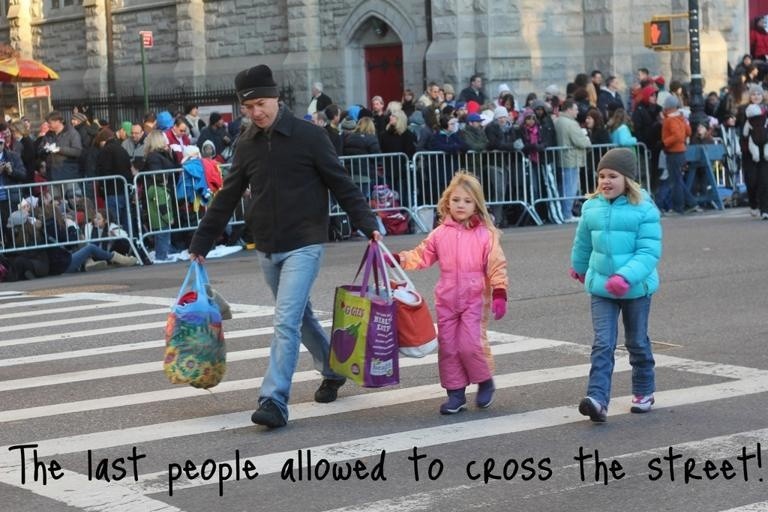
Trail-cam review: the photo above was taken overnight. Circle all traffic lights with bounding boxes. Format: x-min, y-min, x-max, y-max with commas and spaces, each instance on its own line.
643, 20, 673, 49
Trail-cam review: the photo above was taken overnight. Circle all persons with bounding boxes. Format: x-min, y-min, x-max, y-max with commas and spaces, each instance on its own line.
569, 148, 663, 422
375, 172, 510, 415
188, 65, 383, 427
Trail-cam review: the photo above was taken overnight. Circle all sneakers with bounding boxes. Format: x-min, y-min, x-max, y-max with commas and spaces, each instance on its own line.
314, 375, 345, 403
630, 393, 655, 413
579, 396, 607, 423
251, 400, 287, 427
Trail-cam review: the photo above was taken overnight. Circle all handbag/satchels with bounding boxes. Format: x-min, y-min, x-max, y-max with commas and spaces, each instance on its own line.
328, 238, 400, 389
377, 238, 439, 359
163, 256, 227, 390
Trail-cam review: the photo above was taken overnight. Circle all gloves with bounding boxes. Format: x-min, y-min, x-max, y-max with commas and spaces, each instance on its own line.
375, 253, 403, 267
606, 276, 630, 295
490, 291, 507, 318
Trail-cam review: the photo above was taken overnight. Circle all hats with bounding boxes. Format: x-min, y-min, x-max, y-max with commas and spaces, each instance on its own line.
596, 149, 639, 178
234, 64, 280, 103
349, 75, 765, 129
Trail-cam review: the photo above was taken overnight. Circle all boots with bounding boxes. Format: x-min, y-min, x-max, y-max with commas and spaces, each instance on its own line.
439, 388, 468, 415
110, 250, 137, 268
83, 255, 108, 271
476, 377, 496, 408
